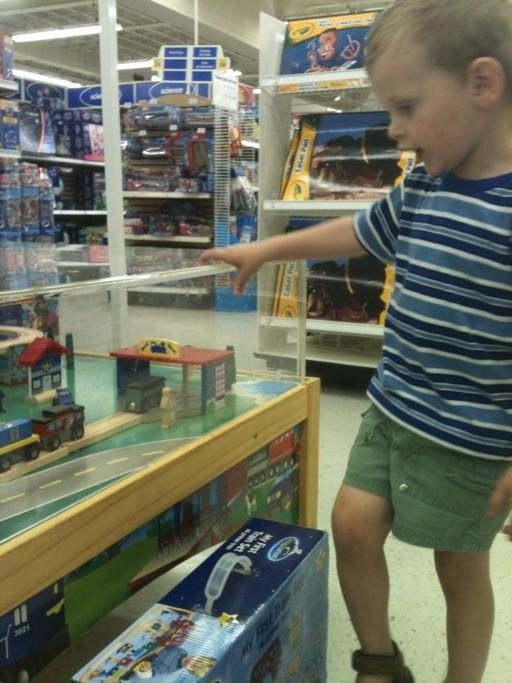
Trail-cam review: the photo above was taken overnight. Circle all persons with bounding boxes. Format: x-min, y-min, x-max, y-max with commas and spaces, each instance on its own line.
197, 0, 511, 681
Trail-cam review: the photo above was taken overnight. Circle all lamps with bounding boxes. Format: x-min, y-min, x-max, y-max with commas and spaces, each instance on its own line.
11, 69, 80, 88
150, 75, 159, 80
234, 70, 242, 76
10, 22, 123, 43
252, 88, 261, 94
116, 59, 155, 70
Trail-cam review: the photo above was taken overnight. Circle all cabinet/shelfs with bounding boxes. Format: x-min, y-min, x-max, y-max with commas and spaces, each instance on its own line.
0, 79, 110, 391
119, 105, 260, 314
253, 10, 385, 369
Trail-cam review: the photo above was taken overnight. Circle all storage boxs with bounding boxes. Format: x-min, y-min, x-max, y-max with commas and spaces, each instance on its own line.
72, 520, 328, 681
